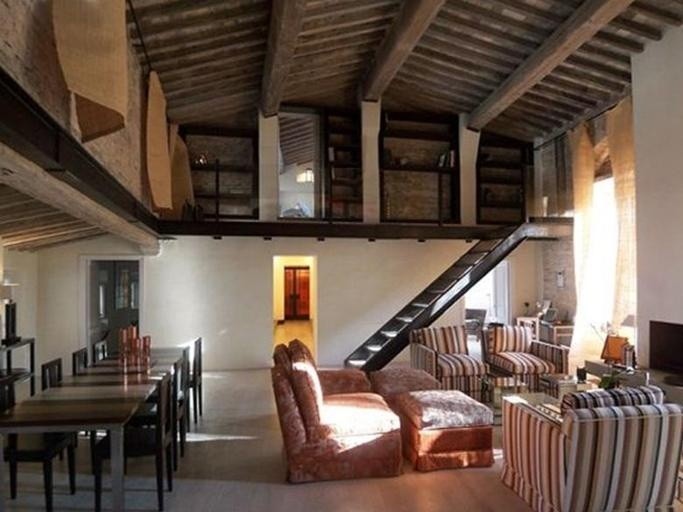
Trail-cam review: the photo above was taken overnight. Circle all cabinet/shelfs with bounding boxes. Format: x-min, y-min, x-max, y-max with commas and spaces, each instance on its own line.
176, 122, 259, 219
322, 108, 527, 225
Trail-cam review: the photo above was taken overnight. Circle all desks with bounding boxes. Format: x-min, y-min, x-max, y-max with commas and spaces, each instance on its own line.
584, 358, 683, 403
514, 316, 574, 345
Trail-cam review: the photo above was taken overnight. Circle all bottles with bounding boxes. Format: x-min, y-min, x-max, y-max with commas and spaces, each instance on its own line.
576, 361, 586, 383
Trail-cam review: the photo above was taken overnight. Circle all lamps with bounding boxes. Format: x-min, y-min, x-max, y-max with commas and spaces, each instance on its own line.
0, 283, 22, 345
601, 334, 628, 364
295, 167, 315, 183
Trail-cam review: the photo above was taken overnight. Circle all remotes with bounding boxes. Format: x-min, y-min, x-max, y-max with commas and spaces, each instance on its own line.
482, 377, 489, 385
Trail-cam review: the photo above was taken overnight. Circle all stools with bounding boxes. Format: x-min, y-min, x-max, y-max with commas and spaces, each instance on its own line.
369, 367, 495, 472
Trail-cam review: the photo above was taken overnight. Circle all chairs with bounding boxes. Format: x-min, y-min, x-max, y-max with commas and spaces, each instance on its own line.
499, 384, 683, 512
407, 308, 571, 403
1, 338, 203, 511
270, 338, 403, 483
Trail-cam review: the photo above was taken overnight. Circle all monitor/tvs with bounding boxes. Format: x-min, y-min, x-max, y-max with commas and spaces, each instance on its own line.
649, 320, 683, 386
